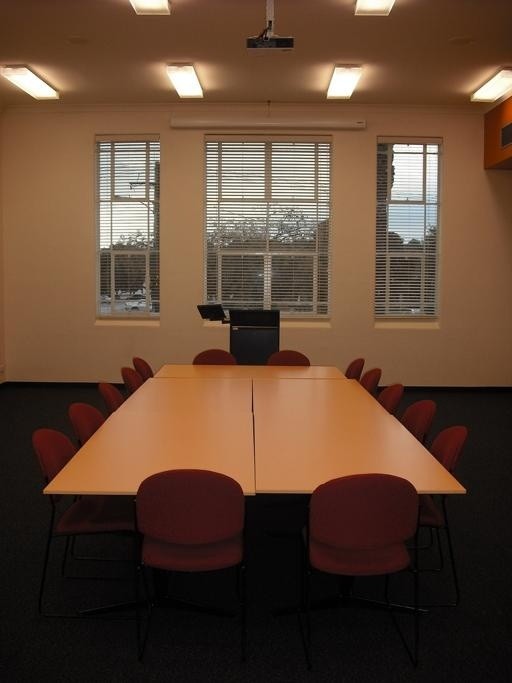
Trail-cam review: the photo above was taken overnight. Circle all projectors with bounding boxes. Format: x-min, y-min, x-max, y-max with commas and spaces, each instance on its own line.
246, 37, 294, 48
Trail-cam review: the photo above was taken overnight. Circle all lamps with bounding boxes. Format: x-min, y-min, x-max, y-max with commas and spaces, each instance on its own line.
0, 64, 58, 101
129, 0, 171, 16
353, 0, 395, 17
167, 64, 203, 99
471, 69, 512, 103
325, 64, 363, 98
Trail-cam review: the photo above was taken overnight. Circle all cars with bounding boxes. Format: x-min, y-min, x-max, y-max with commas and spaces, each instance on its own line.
102, 293, 154, 313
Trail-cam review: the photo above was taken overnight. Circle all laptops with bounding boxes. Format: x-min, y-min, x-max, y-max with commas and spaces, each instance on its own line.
197, 304, 230, 324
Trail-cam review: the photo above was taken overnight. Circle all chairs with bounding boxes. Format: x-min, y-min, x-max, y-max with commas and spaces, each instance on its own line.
264, 350, 310, 366
346, 355, 465, 615
299, 473, 425, 667
194, 349, 236, 365
135, 467, 258, 661
32, 358, 152, 626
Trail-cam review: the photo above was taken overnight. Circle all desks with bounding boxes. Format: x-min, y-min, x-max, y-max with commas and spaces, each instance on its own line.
40, 367, 466, 614
